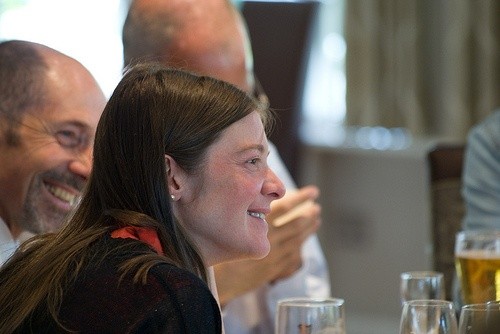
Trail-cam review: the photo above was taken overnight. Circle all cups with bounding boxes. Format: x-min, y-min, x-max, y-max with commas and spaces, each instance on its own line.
398, 299, 459, 334
399, 271, 445, 301
274, 297, 348, 334
454, 230, 500, 308
458, 302, 500, 334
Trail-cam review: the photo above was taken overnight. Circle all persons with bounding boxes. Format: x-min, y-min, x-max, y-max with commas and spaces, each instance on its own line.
0, 67, 285, 334
451, 114, 499, 315
0, 0, 329, 334
